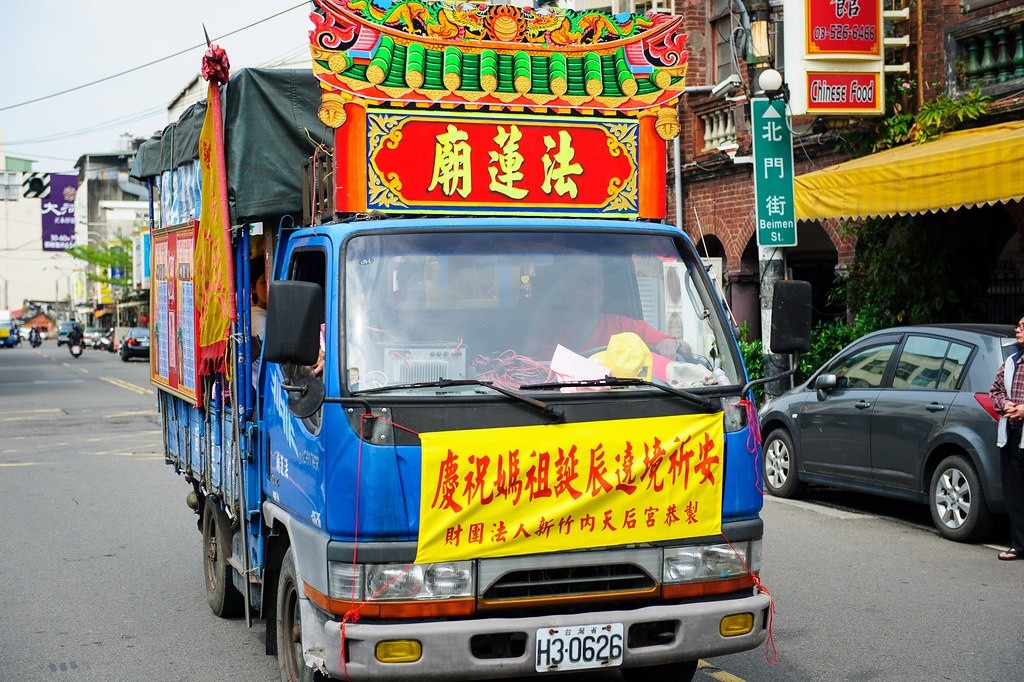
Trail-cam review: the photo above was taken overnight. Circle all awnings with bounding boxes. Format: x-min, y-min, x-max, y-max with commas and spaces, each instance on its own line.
93, 307, 114, 320
773, 115, 1024, 249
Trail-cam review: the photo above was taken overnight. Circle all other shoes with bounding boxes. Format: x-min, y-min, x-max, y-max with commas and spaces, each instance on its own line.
997, 547, 1020, 560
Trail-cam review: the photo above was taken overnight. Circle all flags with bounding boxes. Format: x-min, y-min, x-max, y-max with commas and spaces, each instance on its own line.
195, 91, 234, 374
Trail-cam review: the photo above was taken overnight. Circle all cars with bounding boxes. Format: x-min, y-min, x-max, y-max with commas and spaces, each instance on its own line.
757, 322, 1021, 543
83, 328, 102, 346
19, 326, 50, 340
118, 327, 152, 362
56, 327, 77, 345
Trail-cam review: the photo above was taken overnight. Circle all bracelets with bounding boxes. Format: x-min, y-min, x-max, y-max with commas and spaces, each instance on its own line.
674, 340, 682, 353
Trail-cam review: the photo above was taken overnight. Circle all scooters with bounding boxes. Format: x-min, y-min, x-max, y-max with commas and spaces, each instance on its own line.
66, 335, 85, 359
29, 333, 42, 347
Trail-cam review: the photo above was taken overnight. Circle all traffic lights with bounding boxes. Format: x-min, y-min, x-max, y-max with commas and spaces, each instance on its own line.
23, 172, 52, 199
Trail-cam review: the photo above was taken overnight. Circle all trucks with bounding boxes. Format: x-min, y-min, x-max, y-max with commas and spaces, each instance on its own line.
126, 3, 814, 682
0, 310, 18, 350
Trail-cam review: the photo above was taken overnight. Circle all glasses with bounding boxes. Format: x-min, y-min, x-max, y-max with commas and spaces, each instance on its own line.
1014, 322, 1024, 328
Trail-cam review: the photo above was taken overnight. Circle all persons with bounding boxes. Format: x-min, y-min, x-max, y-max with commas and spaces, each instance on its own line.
312, 270, 408, 393
246, 268, 268, 381
988, 316, 1024, 560
71, 324, 84, 347
514, 251, 694, 389
13, 324, 20, 344
29, 325, 39, 343
